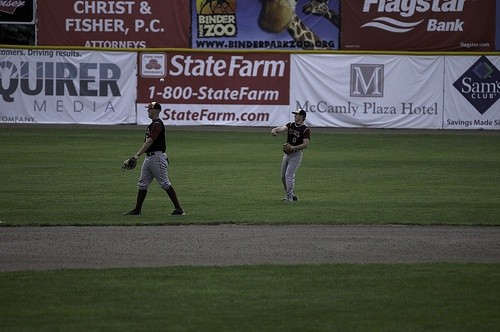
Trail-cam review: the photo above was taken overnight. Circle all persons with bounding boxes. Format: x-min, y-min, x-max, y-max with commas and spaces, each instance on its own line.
120, 102, 185, 215
270, 109, 311, 202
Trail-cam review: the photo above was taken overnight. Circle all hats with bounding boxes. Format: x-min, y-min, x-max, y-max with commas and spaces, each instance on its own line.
292, 109, 306, 117
145, 102, 161, 111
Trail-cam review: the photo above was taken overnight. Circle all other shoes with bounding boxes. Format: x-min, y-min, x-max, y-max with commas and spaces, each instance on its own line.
168, 209, 186, 216
122, 209, 142, 215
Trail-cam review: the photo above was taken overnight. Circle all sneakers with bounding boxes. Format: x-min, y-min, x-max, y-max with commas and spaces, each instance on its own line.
282, 196, 298, 202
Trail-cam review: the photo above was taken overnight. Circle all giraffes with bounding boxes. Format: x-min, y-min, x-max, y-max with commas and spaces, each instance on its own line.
300, 0, 339, 30
253, 0, 333, 50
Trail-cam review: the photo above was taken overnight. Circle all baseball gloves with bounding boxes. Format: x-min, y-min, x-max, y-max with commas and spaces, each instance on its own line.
282, 142, 293, 155
122, 157, 138, 170
